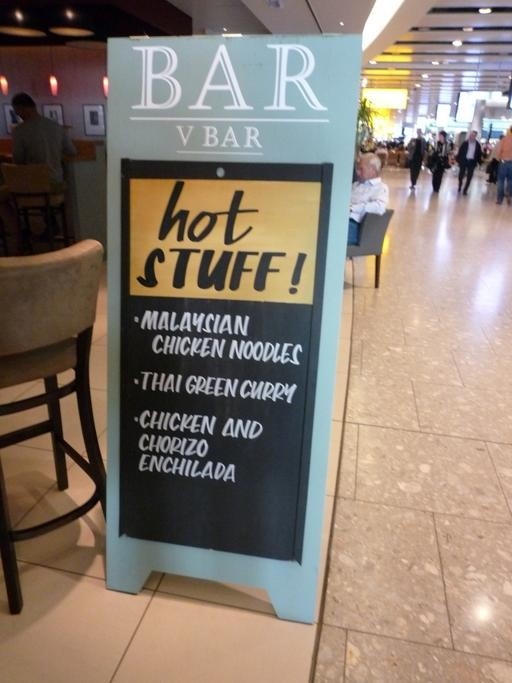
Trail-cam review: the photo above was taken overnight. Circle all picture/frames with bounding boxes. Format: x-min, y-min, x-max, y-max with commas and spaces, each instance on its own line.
2, 102, 18, 135
42, 104, 64, 126
82, 104, 106, 136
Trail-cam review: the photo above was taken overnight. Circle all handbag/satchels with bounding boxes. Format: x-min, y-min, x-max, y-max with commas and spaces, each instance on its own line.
440, 155, 448, 167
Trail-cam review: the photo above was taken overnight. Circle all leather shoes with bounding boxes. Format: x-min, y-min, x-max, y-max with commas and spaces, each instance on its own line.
457, 187, 467, 195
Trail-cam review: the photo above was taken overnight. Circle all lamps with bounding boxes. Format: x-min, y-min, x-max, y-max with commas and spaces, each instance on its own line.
47, 46, 60, 97
0, 75, 9, 96
101, 75, 109, 98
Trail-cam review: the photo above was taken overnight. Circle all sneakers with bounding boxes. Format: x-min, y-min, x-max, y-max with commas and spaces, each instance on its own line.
495, 194, 511, 205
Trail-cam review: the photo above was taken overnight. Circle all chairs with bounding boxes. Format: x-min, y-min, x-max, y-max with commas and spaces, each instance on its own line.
346, 208, 394, 286
1, 237, 105, 615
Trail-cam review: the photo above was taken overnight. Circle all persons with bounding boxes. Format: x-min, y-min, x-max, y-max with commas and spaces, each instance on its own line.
348, 156, 391, 251
9, 90, 78, 241
362, 125, 511, 206
